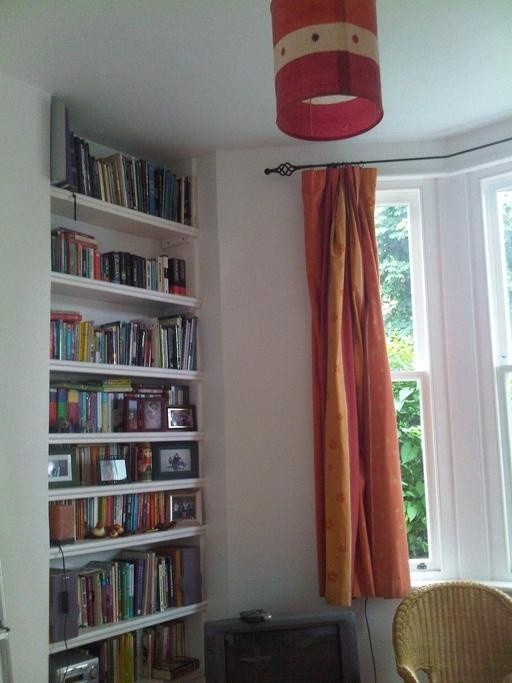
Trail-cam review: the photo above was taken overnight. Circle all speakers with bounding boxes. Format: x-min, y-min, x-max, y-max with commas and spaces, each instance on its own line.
152, 544, 201, 606
50, 568, 77, 644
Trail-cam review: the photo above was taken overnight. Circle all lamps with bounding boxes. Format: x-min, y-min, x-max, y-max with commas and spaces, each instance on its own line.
269, 1, 384, 141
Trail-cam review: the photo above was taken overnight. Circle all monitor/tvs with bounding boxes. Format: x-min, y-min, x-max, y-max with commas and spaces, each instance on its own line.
203, 612, 360, 683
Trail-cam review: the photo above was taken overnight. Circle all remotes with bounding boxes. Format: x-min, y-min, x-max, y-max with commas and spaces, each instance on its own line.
241, 609, 272, 624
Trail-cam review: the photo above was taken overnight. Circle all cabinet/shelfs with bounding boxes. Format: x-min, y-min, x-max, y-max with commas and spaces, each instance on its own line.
48, 103, 204, 683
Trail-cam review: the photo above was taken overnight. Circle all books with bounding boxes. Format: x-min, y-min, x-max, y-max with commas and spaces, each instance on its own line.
49, 378, 189, 433
72, 620, 198, 683
50, 492, 165, 548
50, 546, 198, 643
50, 104, 194, 226
50, 226, 187, 294
49, 443, 153, 488
50, 311, 197, 371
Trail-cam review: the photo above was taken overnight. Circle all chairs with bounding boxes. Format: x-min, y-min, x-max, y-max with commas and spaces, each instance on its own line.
391, 578, 512, 681
